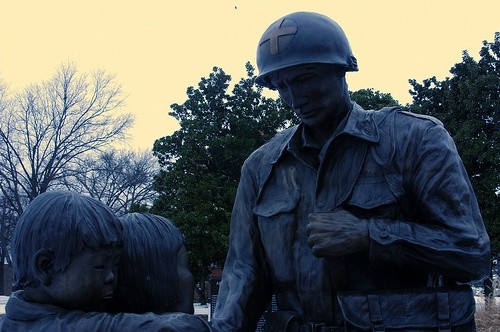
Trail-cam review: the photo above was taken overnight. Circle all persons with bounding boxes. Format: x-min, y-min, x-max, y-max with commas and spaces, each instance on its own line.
117, 212, 196, 314
209, 10, 493, 332
0, 189, 211, 330
